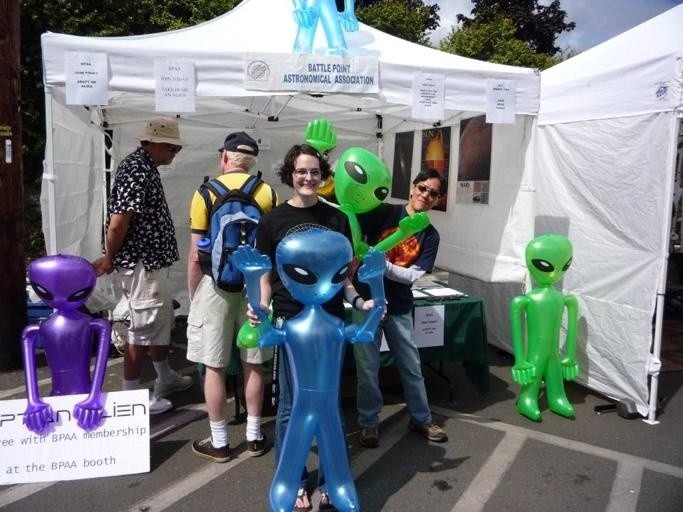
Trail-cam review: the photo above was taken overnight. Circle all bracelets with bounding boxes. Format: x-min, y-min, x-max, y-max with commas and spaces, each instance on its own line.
352, 295, 363, 311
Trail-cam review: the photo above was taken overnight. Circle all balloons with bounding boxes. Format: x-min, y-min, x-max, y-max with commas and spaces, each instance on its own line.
509, 232, 580, 425
21, 253, 109, 431
292, 1, 358, 54
229, 227, 385, 511
236, 118, 429, 350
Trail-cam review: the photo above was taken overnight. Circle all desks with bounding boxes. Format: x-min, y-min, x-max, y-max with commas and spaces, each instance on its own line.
229, 282, 482, 422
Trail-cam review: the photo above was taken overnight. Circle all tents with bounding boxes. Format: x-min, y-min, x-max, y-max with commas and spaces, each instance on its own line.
540, 2, 683, 418
38, 0, 540, 353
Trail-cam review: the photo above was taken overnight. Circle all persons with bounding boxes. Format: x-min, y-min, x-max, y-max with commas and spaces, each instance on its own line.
319, 167, 449, 447
91, 116, 194, 415
187, 132, 278, 462
247, 145, 388, 512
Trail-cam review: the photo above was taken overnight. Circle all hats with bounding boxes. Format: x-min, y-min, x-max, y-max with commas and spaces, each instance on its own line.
217, 131, 260, 157
133, 115, 184, 147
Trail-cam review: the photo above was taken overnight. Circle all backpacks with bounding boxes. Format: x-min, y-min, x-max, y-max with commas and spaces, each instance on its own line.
197, 170, 265, 291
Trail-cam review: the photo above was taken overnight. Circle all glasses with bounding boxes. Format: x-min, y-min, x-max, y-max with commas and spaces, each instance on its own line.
167, 144, 182, 153
416, 183, 439, 197
294, 169, 320, 177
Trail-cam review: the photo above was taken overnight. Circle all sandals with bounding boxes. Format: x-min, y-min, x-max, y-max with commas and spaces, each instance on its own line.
292, 486, 312, 512
318, 492, 337, 512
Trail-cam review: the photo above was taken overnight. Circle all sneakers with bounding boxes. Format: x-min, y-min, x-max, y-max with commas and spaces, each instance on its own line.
408, 416, 447, 442
153, 373, 193, 396
191, 436, 231, 463
358, 426, 380, 447
245, 430, 267, 457
148, 395, 172, 414
